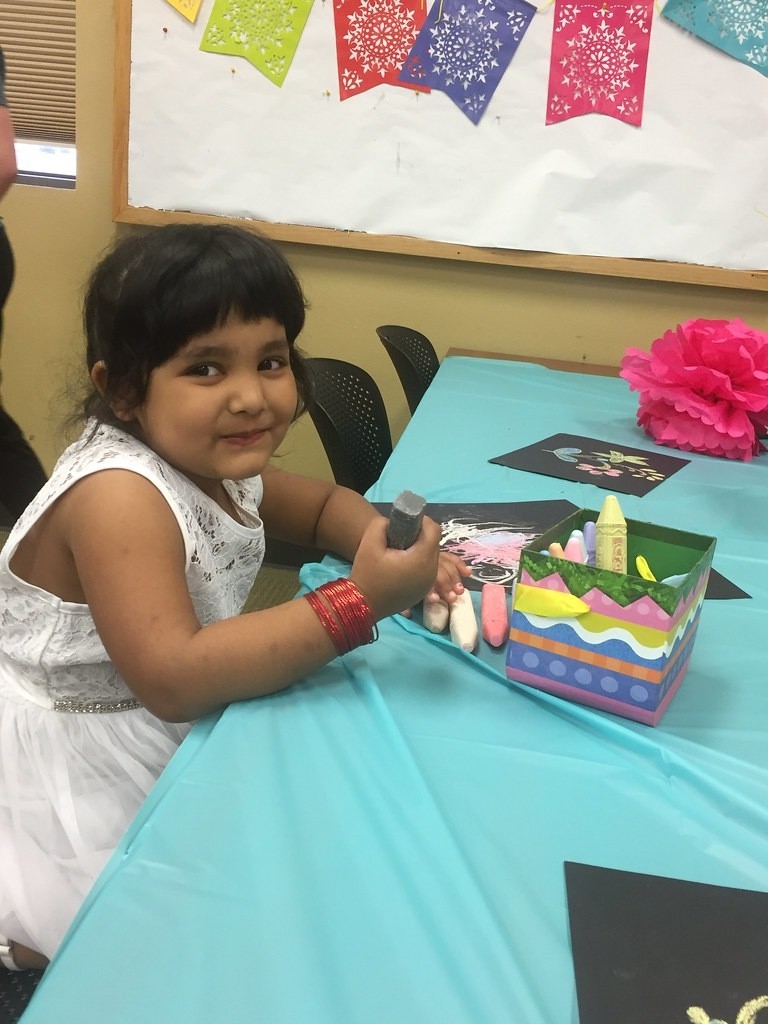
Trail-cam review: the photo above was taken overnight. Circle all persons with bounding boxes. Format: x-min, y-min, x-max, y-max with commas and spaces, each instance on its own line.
0, 46, 50, 537
0, 223, 472, 976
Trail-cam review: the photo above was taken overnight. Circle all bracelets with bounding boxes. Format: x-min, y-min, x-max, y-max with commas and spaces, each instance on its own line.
304, 577, 379, 659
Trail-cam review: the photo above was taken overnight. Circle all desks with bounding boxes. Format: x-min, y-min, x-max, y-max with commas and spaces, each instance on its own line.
18, 348, 768, 1023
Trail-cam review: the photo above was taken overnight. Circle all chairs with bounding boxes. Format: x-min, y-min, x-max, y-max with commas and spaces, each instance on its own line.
375, 323, 440, 417
299, 356, 393, 495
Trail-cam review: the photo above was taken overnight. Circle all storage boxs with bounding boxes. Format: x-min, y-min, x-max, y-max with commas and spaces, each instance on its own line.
506, 507, 719, 730
